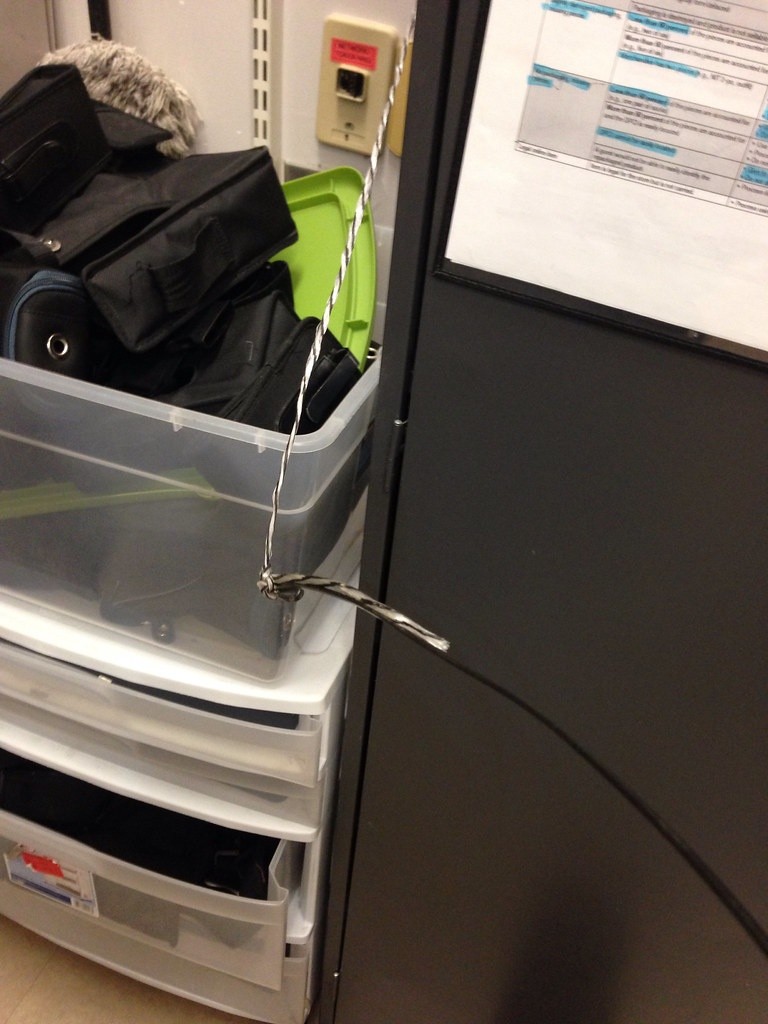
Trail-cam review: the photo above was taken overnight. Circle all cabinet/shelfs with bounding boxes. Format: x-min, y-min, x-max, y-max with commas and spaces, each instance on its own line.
0, 562, 362, 1024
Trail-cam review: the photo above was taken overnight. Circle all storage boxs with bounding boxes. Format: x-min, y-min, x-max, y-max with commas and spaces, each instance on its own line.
1, 166, 383, 683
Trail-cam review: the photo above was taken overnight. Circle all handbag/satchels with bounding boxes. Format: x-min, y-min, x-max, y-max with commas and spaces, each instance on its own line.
0, 64, 380, 660
